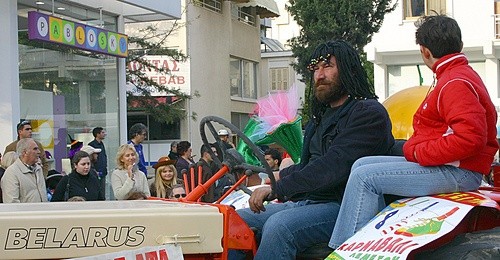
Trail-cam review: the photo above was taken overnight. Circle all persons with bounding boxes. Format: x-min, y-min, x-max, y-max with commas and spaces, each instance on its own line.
49, 150, 106, 202
110, 143, 151, 200
328, 9, 500, 250
0, 138, 49, 203
148, 157, 184, 199
228, 39, 395, 260
0, 120, 294, 203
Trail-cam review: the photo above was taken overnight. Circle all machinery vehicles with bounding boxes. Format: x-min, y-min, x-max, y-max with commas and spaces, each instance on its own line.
0, 116, 500, 259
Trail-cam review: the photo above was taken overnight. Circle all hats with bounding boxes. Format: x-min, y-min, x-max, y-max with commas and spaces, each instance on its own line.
17, 121, 30, 131
80, 145, 101, 156
152, 157, 177, 170
218, 130, 228, 135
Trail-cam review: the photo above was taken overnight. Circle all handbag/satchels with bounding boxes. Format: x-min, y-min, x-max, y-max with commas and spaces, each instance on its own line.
64, 175, 70, 200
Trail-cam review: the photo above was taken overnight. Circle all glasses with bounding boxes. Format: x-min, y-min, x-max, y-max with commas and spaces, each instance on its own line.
26, 128, 32, 130
171, 194, 186, 198
141, 132, 147, 136
